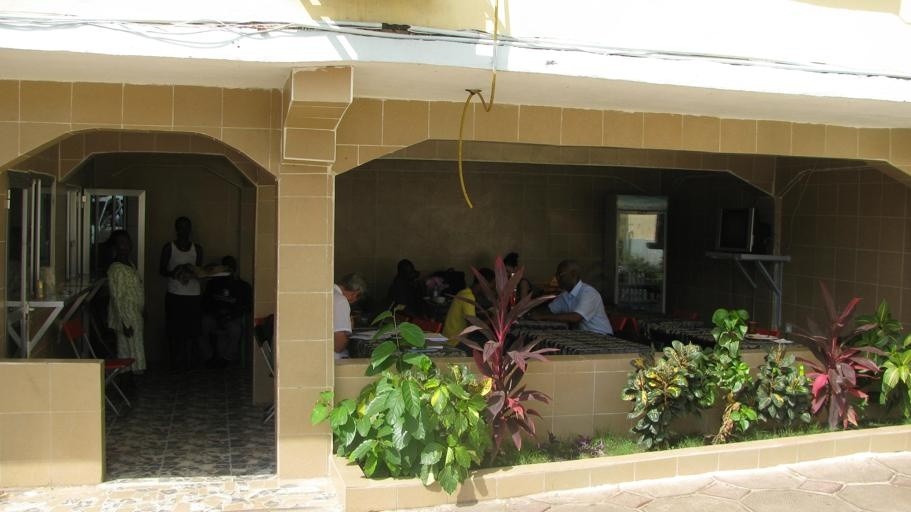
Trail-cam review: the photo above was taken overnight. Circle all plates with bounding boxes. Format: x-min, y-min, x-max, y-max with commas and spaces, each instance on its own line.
745, 334, 777, 341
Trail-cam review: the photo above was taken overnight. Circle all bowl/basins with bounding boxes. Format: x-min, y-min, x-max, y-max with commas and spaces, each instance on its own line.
433, 297, 446, 304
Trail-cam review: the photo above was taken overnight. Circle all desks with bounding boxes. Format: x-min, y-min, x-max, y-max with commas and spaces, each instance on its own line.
344, 306, 786, 354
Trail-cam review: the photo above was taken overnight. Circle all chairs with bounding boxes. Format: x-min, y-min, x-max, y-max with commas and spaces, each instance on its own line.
59, 318, 137, 415
252, 325, 275, 427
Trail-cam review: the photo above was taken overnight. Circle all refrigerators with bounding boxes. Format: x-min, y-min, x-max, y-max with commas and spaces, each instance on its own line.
602, 190, 668, 316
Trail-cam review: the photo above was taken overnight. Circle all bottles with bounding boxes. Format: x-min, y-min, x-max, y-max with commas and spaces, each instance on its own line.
512, 289, 517, 305
618, 267, 662, 309
433, 285, 439, 297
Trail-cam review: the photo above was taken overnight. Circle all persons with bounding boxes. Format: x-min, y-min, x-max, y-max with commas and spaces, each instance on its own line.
160, 217, 204, 363
333, 274, 372, 359
388, 252, 615, 355
103, 230, 147, 376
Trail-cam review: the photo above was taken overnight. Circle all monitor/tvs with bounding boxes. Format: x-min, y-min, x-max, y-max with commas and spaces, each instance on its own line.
713, 204, 766, 256
